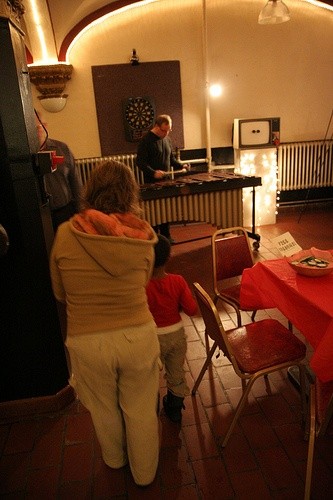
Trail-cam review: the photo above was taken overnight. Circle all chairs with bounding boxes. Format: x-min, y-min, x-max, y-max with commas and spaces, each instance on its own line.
212, 227, 259, 326
191, 280, 308, 451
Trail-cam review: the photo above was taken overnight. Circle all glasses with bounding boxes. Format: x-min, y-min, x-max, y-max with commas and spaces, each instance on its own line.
158, 125, 173, 134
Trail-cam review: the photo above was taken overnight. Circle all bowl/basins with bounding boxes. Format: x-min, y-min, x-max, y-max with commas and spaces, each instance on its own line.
284, 256, 333, 276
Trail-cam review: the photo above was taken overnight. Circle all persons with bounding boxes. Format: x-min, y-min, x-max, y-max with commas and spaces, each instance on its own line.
146, 236, 198, 420
35, 112, 84, 231
50, 161, 161, 484
137, 115, 181, 184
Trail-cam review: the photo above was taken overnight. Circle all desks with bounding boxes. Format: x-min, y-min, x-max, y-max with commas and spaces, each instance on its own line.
134, 169, 262, 250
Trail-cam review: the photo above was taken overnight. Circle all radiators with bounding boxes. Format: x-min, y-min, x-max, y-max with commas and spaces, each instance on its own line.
77, 154, 140, 191
278, 141, 333, 191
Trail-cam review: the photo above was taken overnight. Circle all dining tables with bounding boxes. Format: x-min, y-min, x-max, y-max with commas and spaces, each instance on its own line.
238, 247, 333, 434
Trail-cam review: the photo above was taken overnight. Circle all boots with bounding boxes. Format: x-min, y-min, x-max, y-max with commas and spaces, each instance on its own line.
163, 389, 185, 423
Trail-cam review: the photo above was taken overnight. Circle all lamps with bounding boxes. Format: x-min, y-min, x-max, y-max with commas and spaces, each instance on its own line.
25, 61, 74, 113
257, 0, 291, 25
